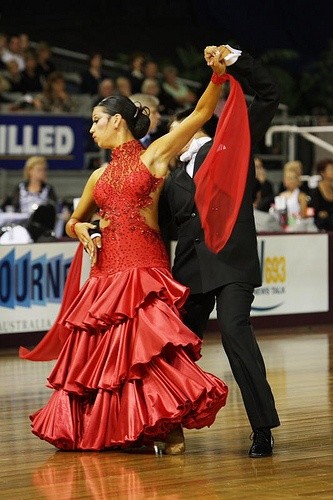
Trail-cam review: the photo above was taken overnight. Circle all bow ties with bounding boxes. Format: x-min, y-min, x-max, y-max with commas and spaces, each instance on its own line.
180, 137, 199, 162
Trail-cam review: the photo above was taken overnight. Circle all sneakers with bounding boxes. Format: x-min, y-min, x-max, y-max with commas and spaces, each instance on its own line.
249, 428, 275, 458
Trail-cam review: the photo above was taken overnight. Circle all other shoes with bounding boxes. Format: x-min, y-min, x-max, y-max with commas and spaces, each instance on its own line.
165, 429, 186, 454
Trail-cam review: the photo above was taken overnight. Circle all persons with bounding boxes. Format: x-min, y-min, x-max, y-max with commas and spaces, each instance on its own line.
84, 45, 281, 459
19, 45, 251, 454
0, 33, 333, 271
